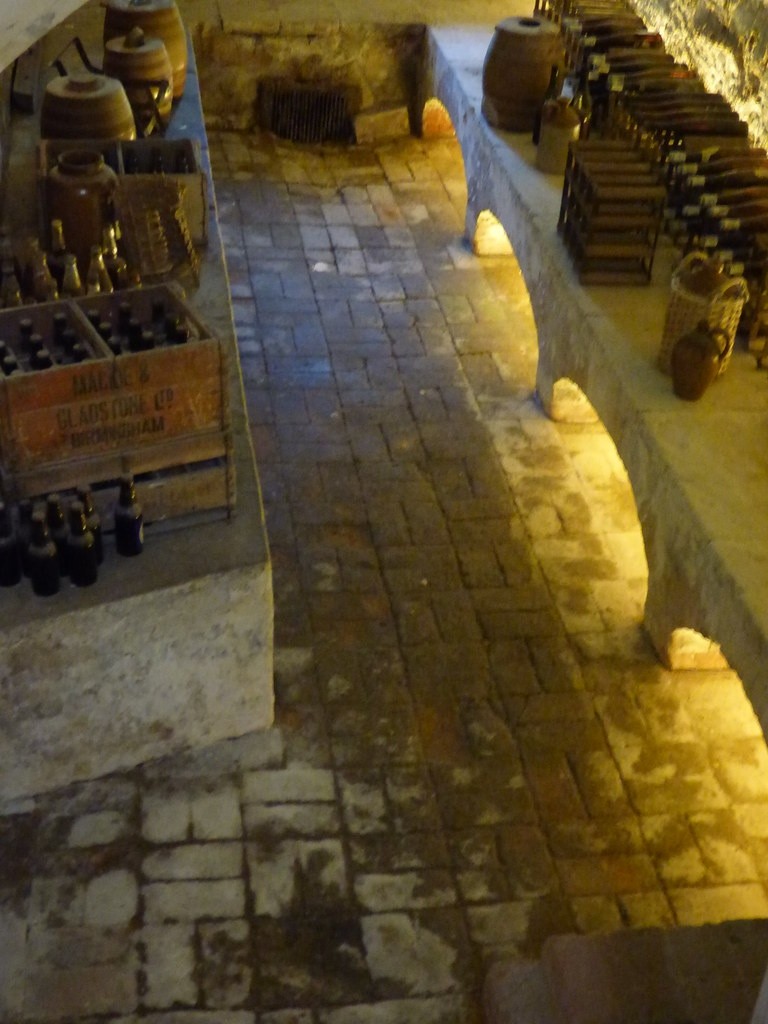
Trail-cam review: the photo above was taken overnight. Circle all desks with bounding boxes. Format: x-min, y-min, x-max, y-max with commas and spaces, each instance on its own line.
0, 0, 91, 143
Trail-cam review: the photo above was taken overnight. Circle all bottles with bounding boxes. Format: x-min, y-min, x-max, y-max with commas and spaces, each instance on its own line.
26, 513, 60, 595
76, 484, 104, 565
15, 500, 39, 577
8, 218, 140, 308
66, 501, 97, 587
532, 65, 560, 144
114, 473, 145, 557
0, 502, 21, 587
45, 494, 73, 577
126, 145, 191, 174
537, 96, 580, 174
670, 319, 730, 401
47, 147, 119, 268
0, 299, 192, 379
563, 13, 768, 296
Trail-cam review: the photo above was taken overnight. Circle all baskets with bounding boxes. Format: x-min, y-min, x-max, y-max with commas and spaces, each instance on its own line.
656, 252, 750, 380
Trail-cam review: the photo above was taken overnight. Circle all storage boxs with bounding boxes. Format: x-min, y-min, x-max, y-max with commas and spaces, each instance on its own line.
0, 282, 227, 497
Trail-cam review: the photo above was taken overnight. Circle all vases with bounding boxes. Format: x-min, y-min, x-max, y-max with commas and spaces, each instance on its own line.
43, 145, 120, 280
